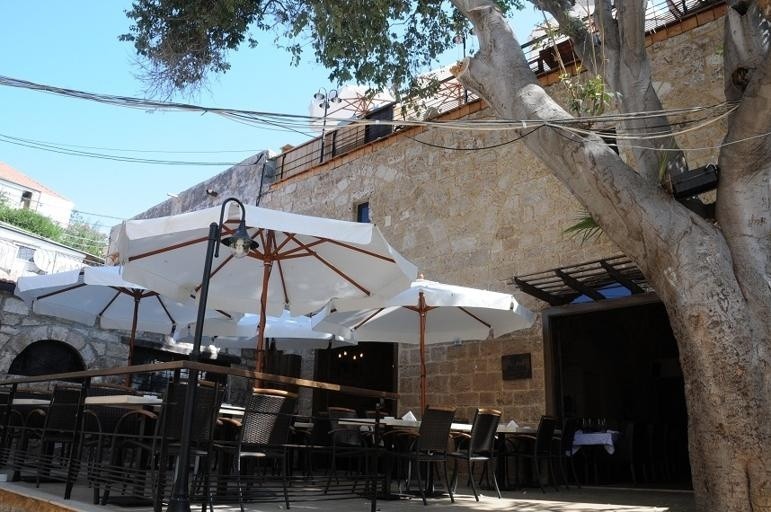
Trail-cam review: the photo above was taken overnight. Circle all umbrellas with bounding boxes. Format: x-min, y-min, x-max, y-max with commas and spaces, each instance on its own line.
9, 265, 246, 388
310, 271, 535, 419
114, 200, 418, 391
212, 308, 359, 369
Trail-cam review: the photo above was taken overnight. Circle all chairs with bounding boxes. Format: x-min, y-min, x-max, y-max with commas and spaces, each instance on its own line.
324, 405, 371, 494
449, 408, 502, 502
2, 383, 33, 482
103, 381, 215, 511
453, 417, 471, 453
505, 413, 559, 494
367, 411, 388, 419
215, 387, 291, 510
381, 403, 455, 506
553, 414, 577, 490
68, 384, 143, 501
8, 383, 83, 488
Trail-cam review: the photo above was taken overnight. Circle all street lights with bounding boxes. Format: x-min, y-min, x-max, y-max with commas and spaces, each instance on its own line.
446, 14, 479, 108
165, 196, 259, 511
313, 85, 343, 165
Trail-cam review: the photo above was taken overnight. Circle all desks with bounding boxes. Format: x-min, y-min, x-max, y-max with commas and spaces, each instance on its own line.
567, 428, 620, 487
